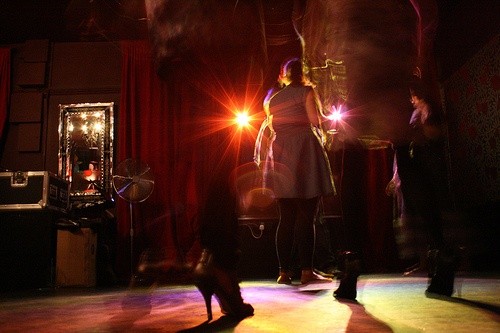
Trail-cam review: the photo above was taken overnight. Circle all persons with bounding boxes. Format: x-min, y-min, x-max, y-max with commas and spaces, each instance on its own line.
193, 56, 466, 320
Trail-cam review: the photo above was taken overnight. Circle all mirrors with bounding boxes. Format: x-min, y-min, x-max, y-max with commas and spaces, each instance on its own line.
58, 102, 115, 205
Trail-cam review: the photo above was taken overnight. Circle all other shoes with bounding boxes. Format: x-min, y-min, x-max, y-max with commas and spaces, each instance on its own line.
300, 271, 333, 284
277, 272, 290, 284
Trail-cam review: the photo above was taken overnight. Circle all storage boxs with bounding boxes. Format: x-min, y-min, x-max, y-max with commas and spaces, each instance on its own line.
0, 170, 71, 212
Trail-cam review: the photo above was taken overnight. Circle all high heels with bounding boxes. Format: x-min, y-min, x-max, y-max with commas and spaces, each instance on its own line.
191, 248, 255, 323
